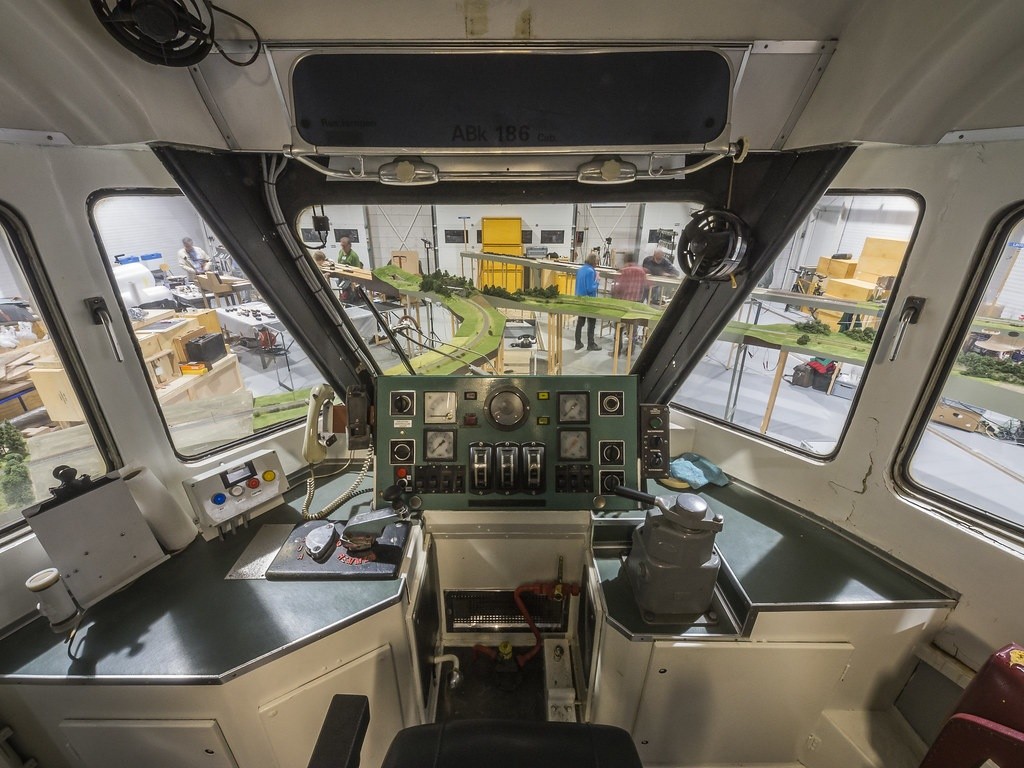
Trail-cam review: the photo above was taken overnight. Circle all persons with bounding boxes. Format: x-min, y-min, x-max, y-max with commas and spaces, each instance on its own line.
337, 237, 360, 269
179, 237, 212, 282
313, 251, 335, 267
608, 252, 649, 358
642, 249, 681, 304
575, 252, 604, 351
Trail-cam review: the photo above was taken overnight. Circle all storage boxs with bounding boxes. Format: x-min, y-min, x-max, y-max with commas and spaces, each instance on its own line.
119, 252, 164, 271
830, 372, 859, 400
801, 237, 909, 332
931, 402, 981, 433
979, 301, 1005, 319
813, 367, 841, 392
29, 309, 244, 429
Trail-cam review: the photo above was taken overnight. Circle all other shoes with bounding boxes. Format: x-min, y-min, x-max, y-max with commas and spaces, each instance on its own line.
587, 342, 602, 351
626, 351, 633, 356
575, 343, 584, 350
608, 351, 621, 357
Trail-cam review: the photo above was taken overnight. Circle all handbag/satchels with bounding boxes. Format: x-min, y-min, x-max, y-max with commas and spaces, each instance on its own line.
782, 357, 838, 392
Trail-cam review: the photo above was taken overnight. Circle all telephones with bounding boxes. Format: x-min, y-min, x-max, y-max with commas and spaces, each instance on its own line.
300, 383, 336, 465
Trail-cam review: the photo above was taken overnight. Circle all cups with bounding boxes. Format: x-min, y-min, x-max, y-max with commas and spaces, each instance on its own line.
25, 568, 86, 635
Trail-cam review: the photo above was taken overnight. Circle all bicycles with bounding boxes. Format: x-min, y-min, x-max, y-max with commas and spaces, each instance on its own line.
784, 268, 829, 317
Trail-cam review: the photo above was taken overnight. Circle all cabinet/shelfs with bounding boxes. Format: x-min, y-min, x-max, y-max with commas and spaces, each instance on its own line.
481, 217, 524, 294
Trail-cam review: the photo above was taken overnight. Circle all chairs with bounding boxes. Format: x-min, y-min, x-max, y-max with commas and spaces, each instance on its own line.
307, 693, 642, 767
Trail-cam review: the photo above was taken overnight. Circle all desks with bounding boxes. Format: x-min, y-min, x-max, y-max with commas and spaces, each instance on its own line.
263, 301, 411, 391
170, 289, 236, 309
215, 301, 279, 369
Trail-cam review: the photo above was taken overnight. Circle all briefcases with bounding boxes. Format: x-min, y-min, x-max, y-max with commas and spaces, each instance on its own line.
173, 326, 207, 363
185, 333, 227, 364
